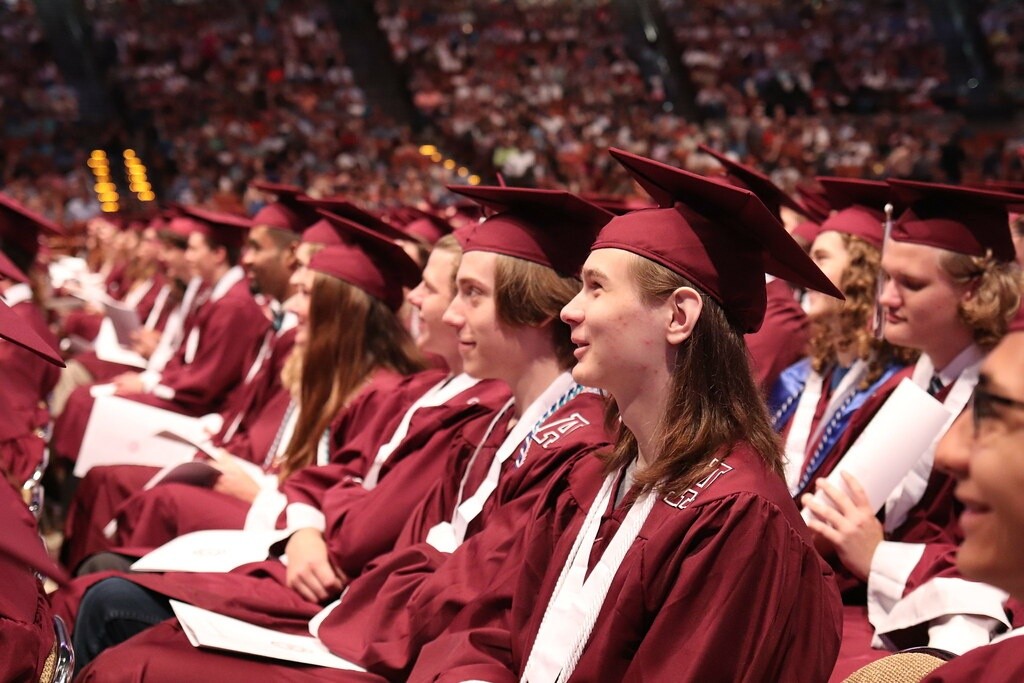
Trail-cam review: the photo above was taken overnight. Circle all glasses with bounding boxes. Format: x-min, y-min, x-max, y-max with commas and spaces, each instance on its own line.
974, 383, 1024, 419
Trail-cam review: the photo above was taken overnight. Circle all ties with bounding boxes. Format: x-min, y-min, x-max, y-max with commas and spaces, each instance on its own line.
927, 376, 943, 395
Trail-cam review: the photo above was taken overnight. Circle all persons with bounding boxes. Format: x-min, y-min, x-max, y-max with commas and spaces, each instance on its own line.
0, 0, 1024, 682
901, 320, 1024, 683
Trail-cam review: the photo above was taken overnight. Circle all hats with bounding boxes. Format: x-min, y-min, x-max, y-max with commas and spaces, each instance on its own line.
0, 192, 69, 251
887, 177, 1024, 262
591, 146, 846, 334
308, 208, 422, 314
183, 207, 253, 248
249, 180, 317, 236
451, 172, 508, 248
445, 184, 617, 277
296, 194, 421, 247
815, 176, 888, 250
697, 144, 822, 226
